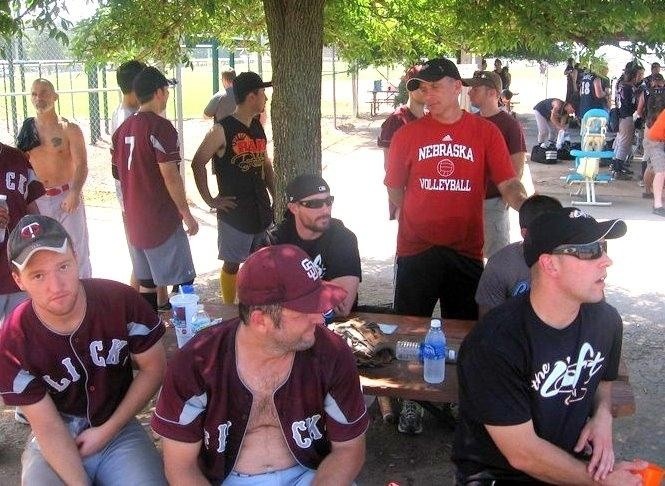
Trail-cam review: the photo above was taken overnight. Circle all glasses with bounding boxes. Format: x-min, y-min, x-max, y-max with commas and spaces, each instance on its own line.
550, 240, 608, 260
298, 196, 334, 208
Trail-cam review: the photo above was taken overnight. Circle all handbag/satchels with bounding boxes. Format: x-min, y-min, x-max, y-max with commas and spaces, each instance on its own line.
531, 141, 557, 164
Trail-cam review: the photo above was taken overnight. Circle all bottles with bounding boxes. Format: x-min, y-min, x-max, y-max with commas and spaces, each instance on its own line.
395, 341, 456, 361
181, 284, 199, 305
424, 319, 445, 383
191, 304, 210, 338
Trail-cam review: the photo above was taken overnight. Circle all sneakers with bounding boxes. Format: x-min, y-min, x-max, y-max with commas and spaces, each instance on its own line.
611, 171, 633, 181
643, 191, 654, 199
397, 400, 424, 435
609, 165, 633, 174
443, 402, 460, 420
653, 206, 665, 217
637, 179, 644, 187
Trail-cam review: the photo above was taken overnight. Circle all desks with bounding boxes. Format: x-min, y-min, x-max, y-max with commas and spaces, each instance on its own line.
124, 297, 638, 433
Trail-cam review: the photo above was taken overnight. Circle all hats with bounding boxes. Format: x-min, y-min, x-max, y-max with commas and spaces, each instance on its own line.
523, 207, 628, 267
406, 58, 461, 91
133, 67, 180, 96
284, 174, 330, 217
235, 242, 349, 315
6, 215, 68, 271
461, 71, 502, 92
233, 71, 273, 96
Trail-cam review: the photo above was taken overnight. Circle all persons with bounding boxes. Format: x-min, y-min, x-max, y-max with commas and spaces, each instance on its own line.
0, 142, 47, 327
0, 214, 168, 485
382, 57, 528, 434
16, 77, 92, 280
635, 66, 645, 80
474, 194, 556, 323
563, 56, 612, 117
252, 171, 362, 317
479, 58, 513, 113
191, 71, 274, 306
461, 70, 525, 265
110, 65, 200, 310
202, 71, 237, 123
609, 68, 638, 181
110, 59, 147, 293
377, 62, 430, 172
641, 102, 665, 218
533, 97, 578, 147
449, 208, 649, 486
633, 73, 665, 187
148, 243, 371, 486
643, 62, 665, 84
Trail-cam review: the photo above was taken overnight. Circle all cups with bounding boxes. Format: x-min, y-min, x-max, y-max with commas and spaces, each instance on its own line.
168, 293, 199, 350
